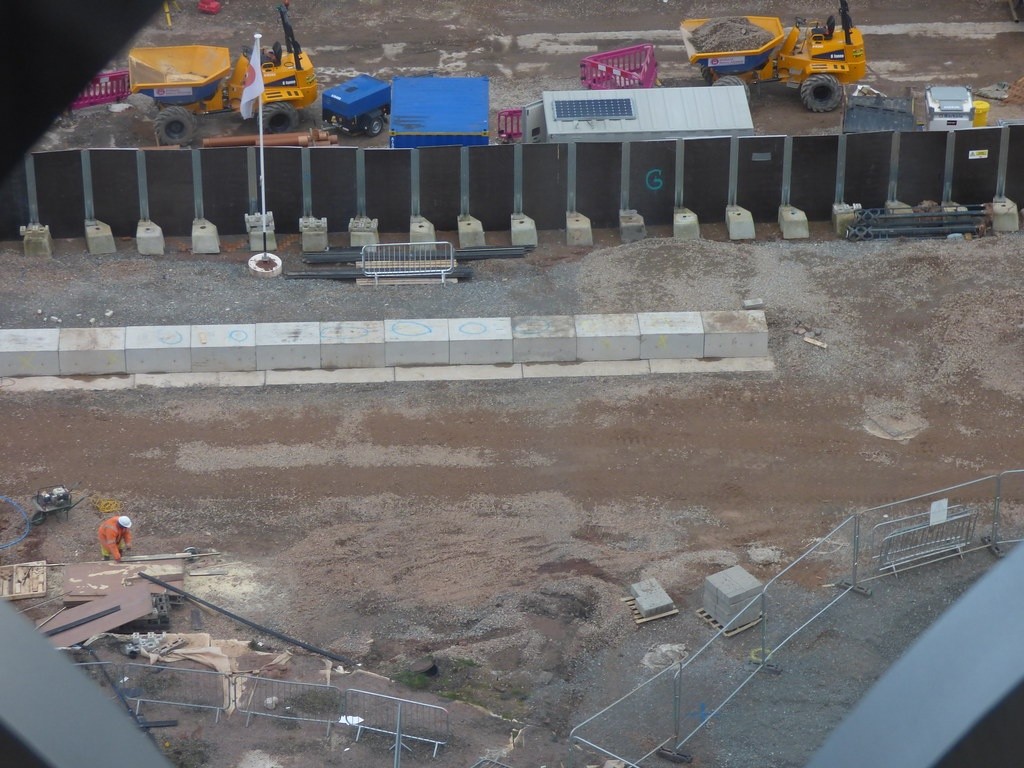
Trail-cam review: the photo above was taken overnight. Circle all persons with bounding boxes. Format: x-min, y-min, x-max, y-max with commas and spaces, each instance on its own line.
99, 516, 131, 561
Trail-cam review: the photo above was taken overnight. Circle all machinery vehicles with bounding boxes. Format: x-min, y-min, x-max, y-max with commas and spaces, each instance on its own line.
678, 0, 867, 112
126, 6, 319, 147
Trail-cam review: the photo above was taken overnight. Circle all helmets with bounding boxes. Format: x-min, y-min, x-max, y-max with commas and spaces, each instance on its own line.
119, 516, 131, 528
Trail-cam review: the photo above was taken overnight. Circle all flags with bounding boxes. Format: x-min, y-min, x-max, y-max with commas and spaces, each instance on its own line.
240, 41, 266, 122
